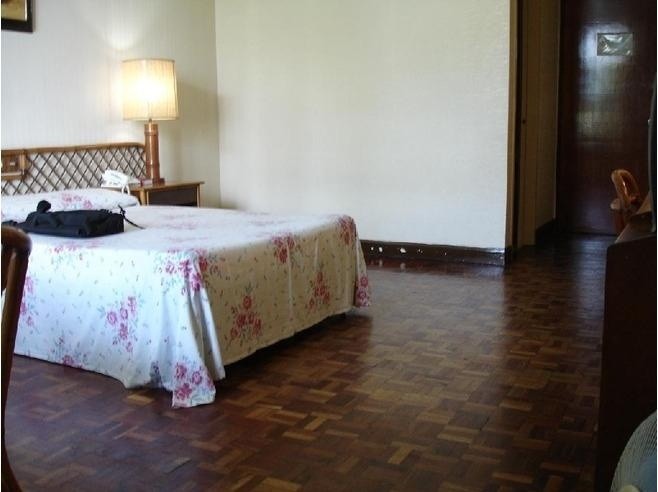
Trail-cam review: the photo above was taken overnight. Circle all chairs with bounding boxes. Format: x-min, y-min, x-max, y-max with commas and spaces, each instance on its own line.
610, 169, 643, 236
1, 227, 32, 492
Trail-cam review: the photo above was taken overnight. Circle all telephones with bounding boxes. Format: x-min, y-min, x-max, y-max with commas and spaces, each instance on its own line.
101, 169, 141, 188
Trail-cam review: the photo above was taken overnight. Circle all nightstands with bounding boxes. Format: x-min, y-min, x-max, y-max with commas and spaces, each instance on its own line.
112, 181, 205, 207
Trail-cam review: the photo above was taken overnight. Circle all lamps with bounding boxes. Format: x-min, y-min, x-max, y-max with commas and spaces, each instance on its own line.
121, 58, 179, 184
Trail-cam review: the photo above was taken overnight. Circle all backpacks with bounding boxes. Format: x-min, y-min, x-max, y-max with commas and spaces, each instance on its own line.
1, 200, 125, 238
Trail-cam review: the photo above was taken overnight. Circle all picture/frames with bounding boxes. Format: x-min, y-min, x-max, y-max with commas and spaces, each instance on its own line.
1, 0, 32, 32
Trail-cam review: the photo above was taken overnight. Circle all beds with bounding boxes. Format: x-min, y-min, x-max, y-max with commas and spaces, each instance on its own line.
1, 142, 352, 393
